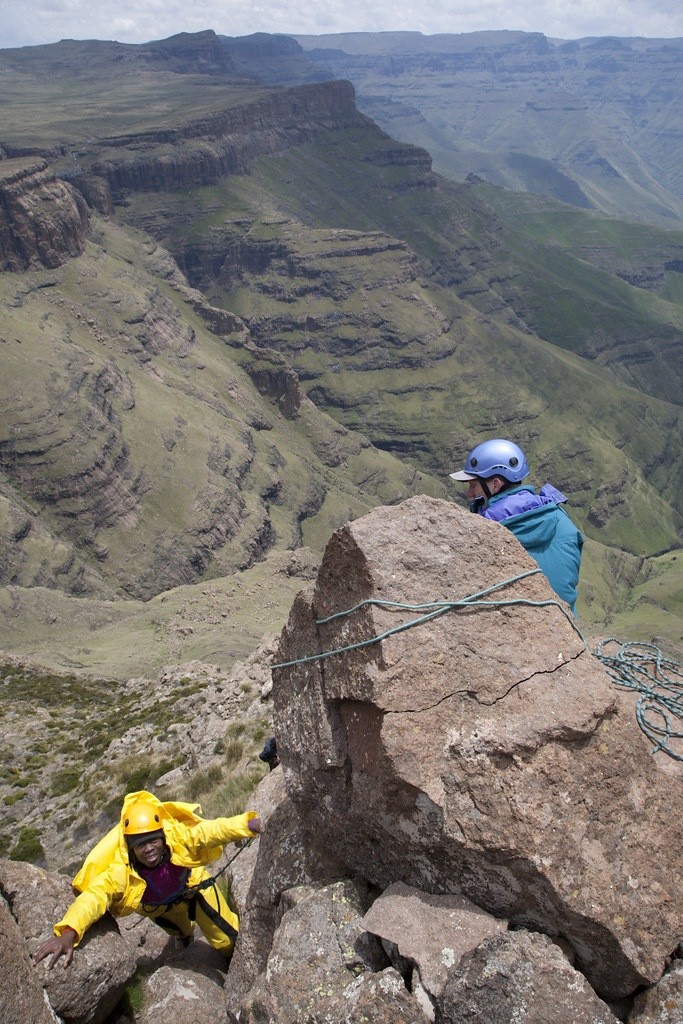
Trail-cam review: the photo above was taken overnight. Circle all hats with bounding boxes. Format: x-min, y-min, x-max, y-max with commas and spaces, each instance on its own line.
127, 830, 163, 849
449, 471, 477, 481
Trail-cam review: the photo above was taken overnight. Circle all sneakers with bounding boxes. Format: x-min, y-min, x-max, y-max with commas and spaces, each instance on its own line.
174, 935, 193, 951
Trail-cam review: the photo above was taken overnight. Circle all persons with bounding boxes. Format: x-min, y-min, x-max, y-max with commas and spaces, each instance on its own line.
33, 790, 261, 970
449, 439, 585, 615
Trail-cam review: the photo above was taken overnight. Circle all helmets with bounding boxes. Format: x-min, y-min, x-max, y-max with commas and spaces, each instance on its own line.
463, 439, 529, 483
121, 802, 163, 835
